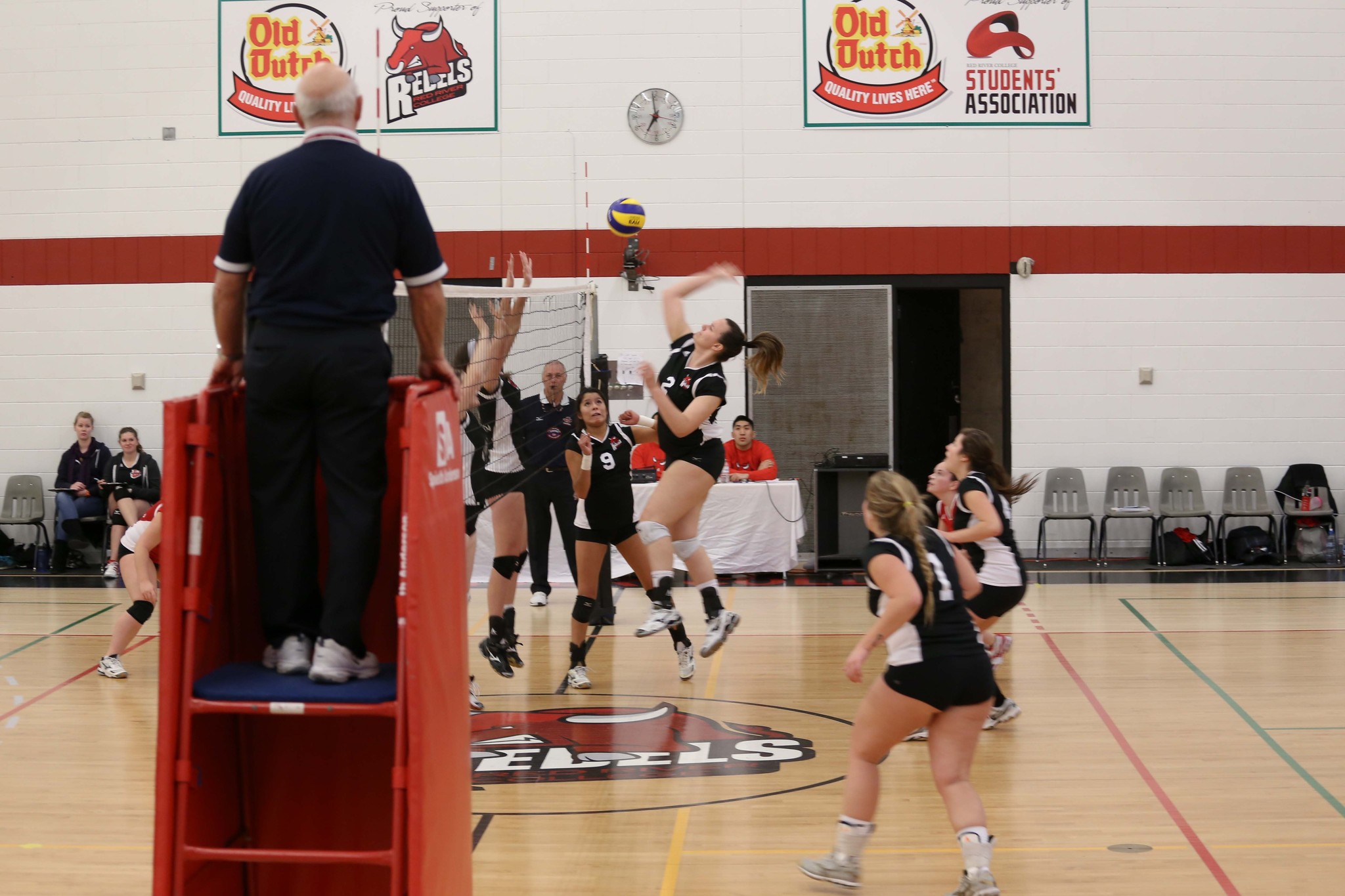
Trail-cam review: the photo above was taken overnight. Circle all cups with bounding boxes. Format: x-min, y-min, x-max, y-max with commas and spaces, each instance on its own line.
1299, 497, 1323, 510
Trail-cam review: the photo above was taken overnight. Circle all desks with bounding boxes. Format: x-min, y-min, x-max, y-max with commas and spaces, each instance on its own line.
451, 477, 807, 582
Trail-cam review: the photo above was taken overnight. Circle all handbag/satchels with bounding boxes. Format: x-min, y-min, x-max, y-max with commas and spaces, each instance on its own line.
1150, 527, 1216, 565
51, 547, 87, 569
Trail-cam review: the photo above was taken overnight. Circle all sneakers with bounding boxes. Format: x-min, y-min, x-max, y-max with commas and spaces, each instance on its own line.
567, 666, 593, 689
635, 601, 682, 638
950, 867, 1001, 896
469, 675, 484, 711
900, 723, 930, 741
673, 638, 695, 681
530, 591, 548, 607
479, 633, 524, 679
104, 561, 122, 578
796, 847, 864, 886
261, 633, 312, 673
981, 697, 1022, 730
307, 638, 381, 683
700, 608, 740, 658
97, 654, 128, 679
983, 633, 1013, 668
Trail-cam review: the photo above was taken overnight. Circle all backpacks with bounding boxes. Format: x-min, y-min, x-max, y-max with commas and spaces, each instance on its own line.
1292, 518, 1335, 563
1227, 525, 1285, 565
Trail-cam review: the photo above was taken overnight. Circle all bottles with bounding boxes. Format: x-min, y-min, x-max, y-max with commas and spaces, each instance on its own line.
37, 546, 47, 573
1343, 543, 1345, 565
1326, 528, 1336, 564
1301, 484, 1313, 511
719, 459, 730, 483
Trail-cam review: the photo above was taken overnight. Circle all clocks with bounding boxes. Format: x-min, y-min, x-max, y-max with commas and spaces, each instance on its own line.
625, 88, 682, 145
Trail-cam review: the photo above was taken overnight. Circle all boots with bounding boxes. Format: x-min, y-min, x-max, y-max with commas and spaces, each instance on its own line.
49, 539, 68, 573
62, 517, 90, 549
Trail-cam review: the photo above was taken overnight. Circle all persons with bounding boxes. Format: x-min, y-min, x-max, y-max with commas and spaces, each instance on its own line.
448, 252, 1042, 896
208, 62, 465, 684
54, 412, 164, 680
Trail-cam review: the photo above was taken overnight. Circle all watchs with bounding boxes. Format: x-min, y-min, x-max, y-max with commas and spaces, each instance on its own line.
217, 345, 242, 361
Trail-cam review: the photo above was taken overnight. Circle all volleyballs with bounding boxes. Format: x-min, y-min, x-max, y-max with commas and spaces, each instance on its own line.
607, 197, 645, 238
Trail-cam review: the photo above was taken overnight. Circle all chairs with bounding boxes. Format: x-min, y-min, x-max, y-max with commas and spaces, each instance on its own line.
0, 475, 131, 572
1035, 466, 1338, 569
